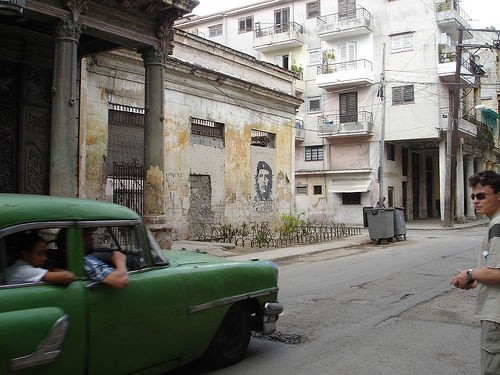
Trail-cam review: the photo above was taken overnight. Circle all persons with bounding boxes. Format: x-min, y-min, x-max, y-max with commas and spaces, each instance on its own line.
451, 171, 500, 375
2, 236, 74, 284
56, 226, 127, 288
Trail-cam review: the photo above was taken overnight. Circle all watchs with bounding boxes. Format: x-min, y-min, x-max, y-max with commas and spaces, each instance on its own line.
465, 268, 475, 283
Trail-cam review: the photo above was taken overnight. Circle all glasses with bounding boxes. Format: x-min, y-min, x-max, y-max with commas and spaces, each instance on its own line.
471, 192, 493, 200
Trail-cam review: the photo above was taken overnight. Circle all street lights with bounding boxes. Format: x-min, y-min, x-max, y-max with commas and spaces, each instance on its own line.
450, 96, 486, 224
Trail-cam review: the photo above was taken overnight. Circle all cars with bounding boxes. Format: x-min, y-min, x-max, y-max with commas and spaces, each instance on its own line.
0, 194, 283, 375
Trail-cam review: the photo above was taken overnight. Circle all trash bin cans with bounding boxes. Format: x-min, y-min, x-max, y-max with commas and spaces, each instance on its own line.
363, 206, 407, 245
